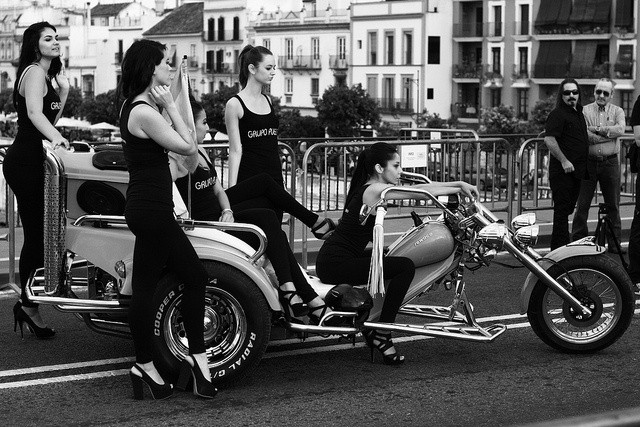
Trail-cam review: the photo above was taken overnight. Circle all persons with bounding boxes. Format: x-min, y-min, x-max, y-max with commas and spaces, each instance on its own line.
2, 22, 70, 337
317, 142, 480, 364
168, 101, 343, 325
629, 95, 640, 289
544, 78, 589, 250
119, 40, 218, 402
224, 45, 284, 228
225, 173, 340, 240
573, 78, 626, 254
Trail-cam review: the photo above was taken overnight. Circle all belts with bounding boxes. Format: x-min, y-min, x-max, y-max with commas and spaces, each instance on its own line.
589, 154, 617, 161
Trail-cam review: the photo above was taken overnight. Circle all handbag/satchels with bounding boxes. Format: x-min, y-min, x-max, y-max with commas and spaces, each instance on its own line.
323, 285, 374, 327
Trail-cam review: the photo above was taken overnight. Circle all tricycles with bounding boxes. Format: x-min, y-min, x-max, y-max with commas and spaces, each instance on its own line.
37, 139, 636, 384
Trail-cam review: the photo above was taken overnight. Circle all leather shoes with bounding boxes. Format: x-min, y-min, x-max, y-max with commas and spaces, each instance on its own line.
608, 246, 627, 254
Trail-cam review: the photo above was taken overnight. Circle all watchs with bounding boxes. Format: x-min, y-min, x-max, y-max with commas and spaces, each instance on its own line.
595, 126, 600, 135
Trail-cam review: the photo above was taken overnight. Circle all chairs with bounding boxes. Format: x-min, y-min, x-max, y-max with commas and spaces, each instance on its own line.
92, 149, 279, 286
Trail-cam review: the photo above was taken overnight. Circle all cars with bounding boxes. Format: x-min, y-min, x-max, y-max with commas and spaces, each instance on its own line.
445, 166, 508, 190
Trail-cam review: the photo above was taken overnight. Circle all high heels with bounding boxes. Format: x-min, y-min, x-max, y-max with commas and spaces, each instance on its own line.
306, 305, 346, 328
13, 300, 34, 335
175, 354, 218, 399
368, 330, 407, 365
128, 362, 174, 402
310, 218, 343, 240
278, 286, 310, 319
17, 305, 55, 339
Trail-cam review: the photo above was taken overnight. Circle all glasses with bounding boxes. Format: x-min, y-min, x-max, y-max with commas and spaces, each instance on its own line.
562, 90, 579, 96
595, 89, 610, 97
